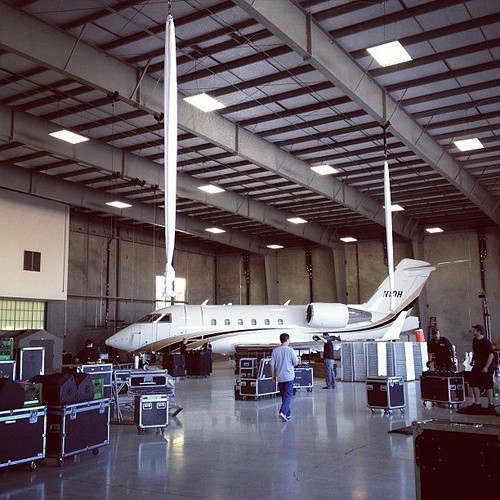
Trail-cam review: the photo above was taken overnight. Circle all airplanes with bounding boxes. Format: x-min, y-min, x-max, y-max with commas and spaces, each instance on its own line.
104, 257, 437, 357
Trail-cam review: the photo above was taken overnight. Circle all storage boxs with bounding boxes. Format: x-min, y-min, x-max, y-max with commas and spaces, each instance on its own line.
239, 357, 314, 400
419, 373, 466, 410
365, 375, 406, 414
0, 345, 171, 470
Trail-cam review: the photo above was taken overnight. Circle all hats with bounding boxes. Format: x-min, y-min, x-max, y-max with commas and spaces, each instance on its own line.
472, 325, 484, 332
432, 327, 439, 332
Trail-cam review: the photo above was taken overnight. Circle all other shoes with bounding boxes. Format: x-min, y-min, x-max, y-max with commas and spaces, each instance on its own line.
287, 416, 292, 419
487, 405, 495, 410
471, 403, 482, 407
279, 412, 288, 423
332, 386, 336, 388
323, 387, 330, 389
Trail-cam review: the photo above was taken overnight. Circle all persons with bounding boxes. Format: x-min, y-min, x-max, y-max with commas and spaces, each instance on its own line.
469, 325, 497, 410
428, 328, 455, 373
312, 333, 336, 389
181, 338, 212, 379
270, 333, 299, 422
75, 340, 101, 364
